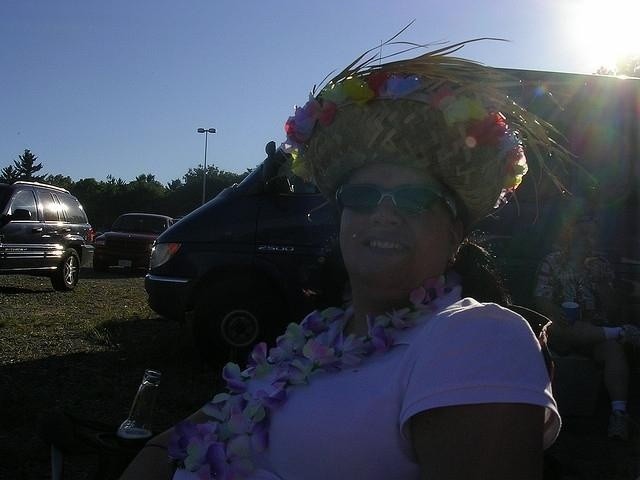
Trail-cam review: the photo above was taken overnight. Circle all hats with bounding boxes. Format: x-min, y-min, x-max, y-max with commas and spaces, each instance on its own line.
280, 75, 528, 231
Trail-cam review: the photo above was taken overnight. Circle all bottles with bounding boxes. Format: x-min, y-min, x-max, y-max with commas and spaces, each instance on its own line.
117, 367, 163, 448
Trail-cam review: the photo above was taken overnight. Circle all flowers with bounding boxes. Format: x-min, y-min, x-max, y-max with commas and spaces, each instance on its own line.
283, 72, 529, 212
164, 277, 463, 480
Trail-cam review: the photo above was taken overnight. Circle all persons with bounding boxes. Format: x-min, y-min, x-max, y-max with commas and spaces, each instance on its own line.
531, 211, 640, 441
118, 26, 598, 480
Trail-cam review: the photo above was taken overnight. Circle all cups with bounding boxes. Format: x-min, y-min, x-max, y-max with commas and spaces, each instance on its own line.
561, 300, 580, 321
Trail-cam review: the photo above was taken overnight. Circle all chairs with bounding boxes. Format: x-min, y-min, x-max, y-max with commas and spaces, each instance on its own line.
509, 300, 555, 394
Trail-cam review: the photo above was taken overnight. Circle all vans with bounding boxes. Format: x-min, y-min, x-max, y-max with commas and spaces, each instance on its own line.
145, 67, 639, 367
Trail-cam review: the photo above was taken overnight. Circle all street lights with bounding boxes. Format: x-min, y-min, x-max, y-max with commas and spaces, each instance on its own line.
198, 126, 217, 205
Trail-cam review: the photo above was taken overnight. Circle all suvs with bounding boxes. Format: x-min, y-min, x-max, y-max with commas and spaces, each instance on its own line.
0, 178, 99, 290
94, 212, 180, 272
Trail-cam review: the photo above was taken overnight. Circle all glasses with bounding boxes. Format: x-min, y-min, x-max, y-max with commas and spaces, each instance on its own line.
335, 182, 457, 220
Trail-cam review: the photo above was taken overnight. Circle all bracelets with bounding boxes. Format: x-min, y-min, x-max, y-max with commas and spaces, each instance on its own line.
143, 439, 168, 452
